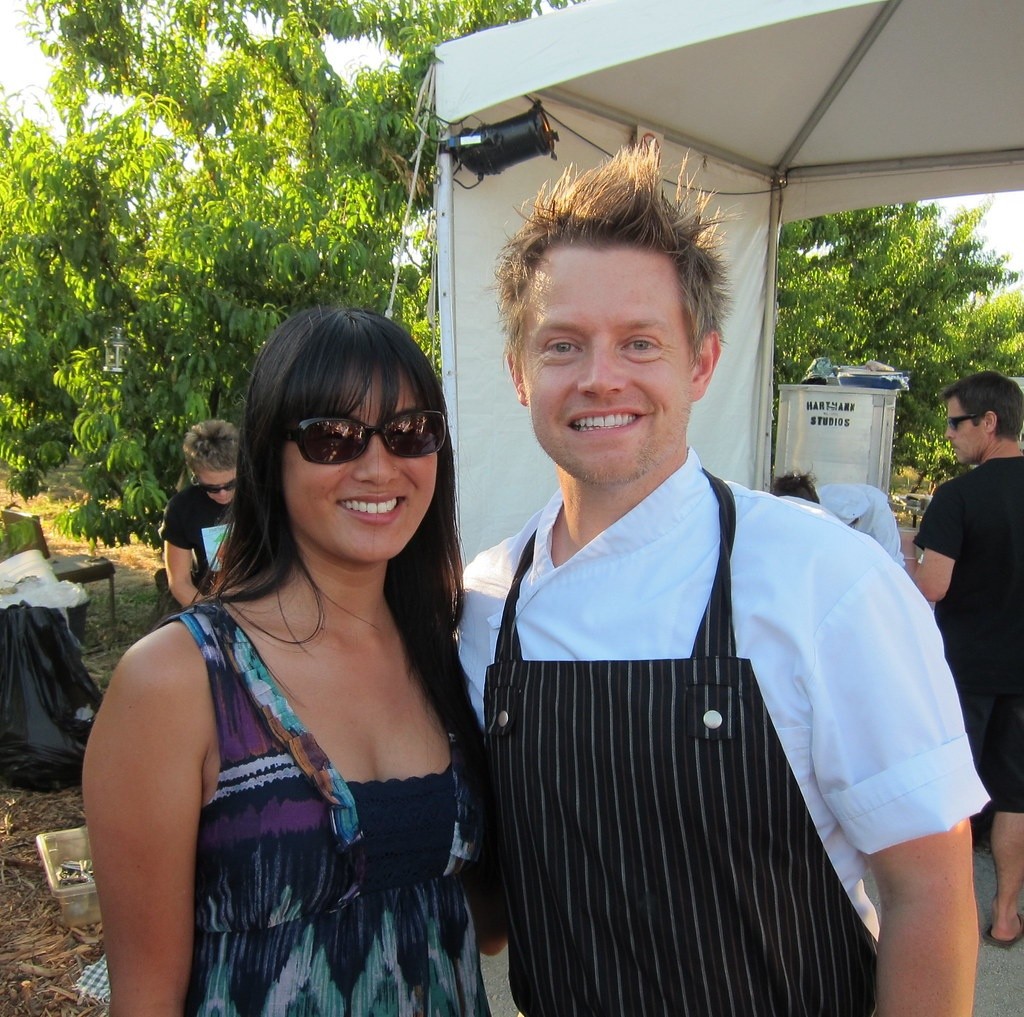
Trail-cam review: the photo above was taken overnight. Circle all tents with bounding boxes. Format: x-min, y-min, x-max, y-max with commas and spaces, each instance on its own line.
434, 0, 1024, 572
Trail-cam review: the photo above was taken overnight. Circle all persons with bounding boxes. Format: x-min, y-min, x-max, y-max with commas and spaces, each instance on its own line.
81, 307, 490, 1017
772, 474, 905, 569
458, 140, 991, 1017
157, 419, 237, 606
901, 371, 1024, 947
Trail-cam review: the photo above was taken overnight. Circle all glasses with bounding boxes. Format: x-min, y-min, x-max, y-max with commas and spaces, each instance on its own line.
947, 414, 977, 430
285, 410, 446, 464
199, 476, 236, 493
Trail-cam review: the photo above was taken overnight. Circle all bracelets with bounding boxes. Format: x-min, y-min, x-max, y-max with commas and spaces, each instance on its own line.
904, 558, 917, 560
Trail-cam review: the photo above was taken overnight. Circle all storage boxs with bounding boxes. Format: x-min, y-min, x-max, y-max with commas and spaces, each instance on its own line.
36, 824, 103, 927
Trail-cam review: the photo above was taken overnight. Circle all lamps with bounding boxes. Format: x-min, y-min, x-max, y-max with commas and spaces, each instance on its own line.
438, 97, 560, 189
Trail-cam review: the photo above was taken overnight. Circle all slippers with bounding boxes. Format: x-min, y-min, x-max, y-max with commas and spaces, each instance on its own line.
983, 913, 1024, 948
